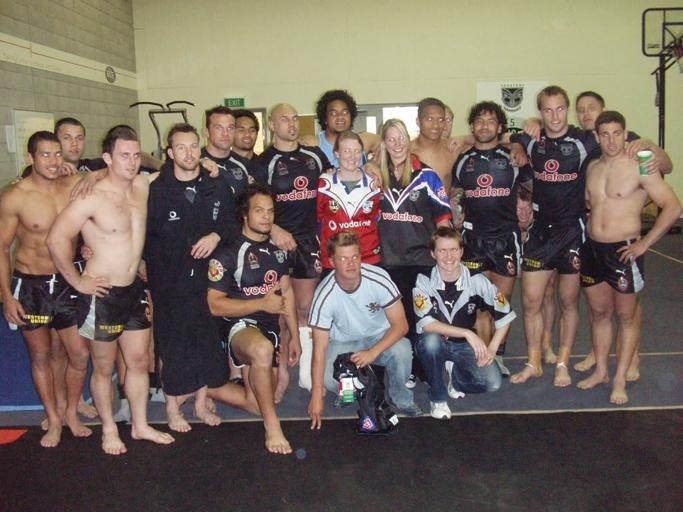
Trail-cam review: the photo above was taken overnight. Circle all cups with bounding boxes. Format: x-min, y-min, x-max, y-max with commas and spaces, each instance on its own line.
637, 151, 652, 177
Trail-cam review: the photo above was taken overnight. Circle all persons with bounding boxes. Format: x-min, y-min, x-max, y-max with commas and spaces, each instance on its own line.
1, 84, 683, 460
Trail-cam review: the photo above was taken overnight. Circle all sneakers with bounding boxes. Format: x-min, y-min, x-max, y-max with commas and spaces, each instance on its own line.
404, 374, 416, 388
388, 398, 423, 417
333, 394, 351, 407
429, 399, 452, 419
494, 355, 510, 377
444, 360, 465, 399
